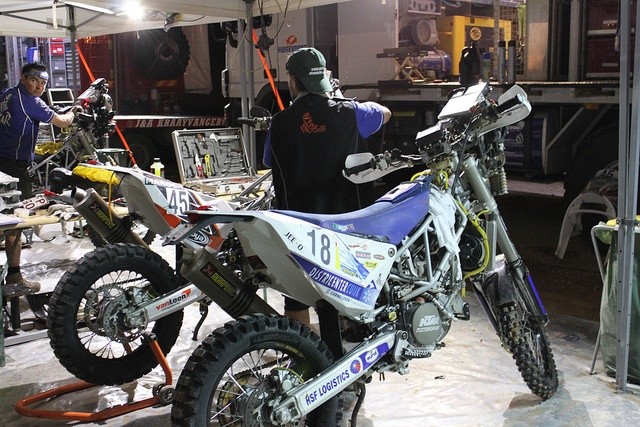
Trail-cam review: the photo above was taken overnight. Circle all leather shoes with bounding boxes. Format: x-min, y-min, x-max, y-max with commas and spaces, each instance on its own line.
5, 273, 40, 293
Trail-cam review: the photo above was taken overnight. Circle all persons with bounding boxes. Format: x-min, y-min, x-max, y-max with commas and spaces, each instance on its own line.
0, 63, 83, 292
263, 48, 392, 327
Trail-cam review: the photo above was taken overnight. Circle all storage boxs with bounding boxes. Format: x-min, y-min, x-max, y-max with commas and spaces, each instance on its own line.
585, 35, 635, 77
587, 0, 637, 35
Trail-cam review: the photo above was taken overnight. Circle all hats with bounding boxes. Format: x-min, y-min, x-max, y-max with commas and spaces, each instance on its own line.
285, 47, 333, 93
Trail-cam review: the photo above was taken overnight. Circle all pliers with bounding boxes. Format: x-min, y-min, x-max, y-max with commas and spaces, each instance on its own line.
193, 154, 204, 178
210, 133, 241, 141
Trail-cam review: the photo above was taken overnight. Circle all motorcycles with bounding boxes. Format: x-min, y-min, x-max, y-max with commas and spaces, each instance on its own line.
46, 125, 379, 386
163, 41, 559, 427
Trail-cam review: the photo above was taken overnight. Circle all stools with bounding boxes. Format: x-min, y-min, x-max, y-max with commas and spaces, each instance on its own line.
376, 52, 427, 83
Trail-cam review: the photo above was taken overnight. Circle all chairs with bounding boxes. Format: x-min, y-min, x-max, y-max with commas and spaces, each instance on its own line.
588, 226, 614, 374
